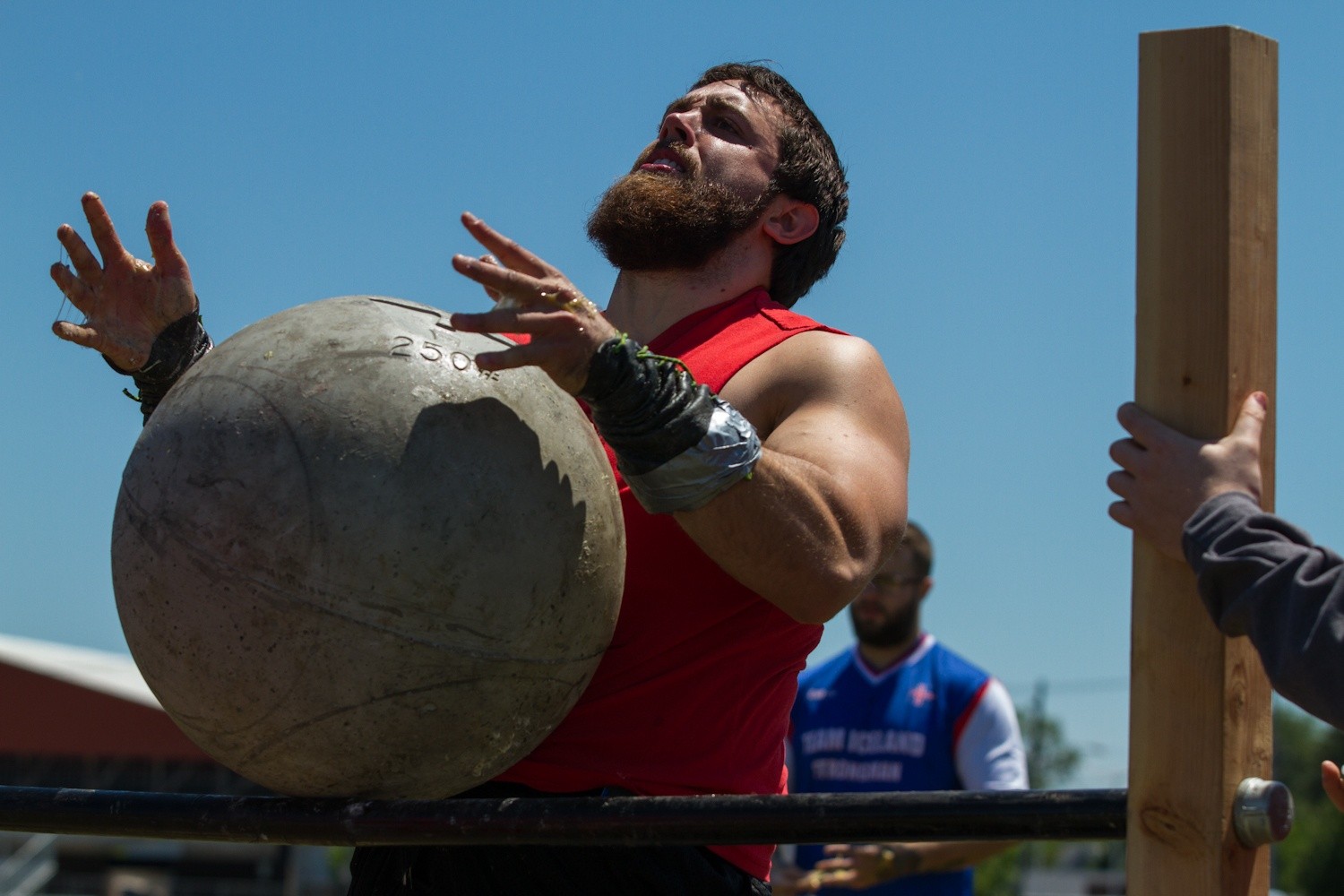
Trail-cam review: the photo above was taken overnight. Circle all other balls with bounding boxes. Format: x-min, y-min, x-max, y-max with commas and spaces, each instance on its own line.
110, 294, 628, 799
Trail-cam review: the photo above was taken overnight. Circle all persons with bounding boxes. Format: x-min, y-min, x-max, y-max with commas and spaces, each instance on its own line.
1106, 391, 1344, 814
774, 524, 1029, 896
50, 64, 911, 896
